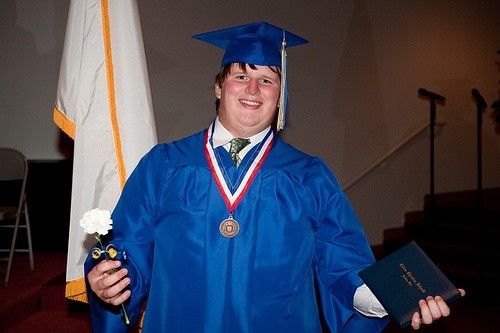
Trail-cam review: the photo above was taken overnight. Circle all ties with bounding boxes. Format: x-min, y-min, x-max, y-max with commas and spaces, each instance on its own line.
227, 137, 251, 168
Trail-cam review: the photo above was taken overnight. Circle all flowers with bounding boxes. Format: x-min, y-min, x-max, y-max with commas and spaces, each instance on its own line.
79, 209, 132, 325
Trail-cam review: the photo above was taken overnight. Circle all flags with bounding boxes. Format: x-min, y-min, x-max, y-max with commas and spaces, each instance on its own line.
54, 1, 158, 304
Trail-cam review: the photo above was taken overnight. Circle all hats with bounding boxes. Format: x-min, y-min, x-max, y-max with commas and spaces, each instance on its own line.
191, 20, 310, 132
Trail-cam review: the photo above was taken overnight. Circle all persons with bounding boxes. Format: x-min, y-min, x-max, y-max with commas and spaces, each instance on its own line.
84, 22, 465, 333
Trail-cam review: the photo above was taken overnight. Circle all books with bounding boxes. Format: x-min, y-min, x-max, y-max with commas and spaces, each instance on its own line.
359, 242, 459, 327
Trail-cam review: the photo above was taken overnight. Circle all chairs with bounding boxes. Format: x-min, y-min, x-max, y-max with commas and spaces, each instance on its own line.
0, 147, 35, 289
